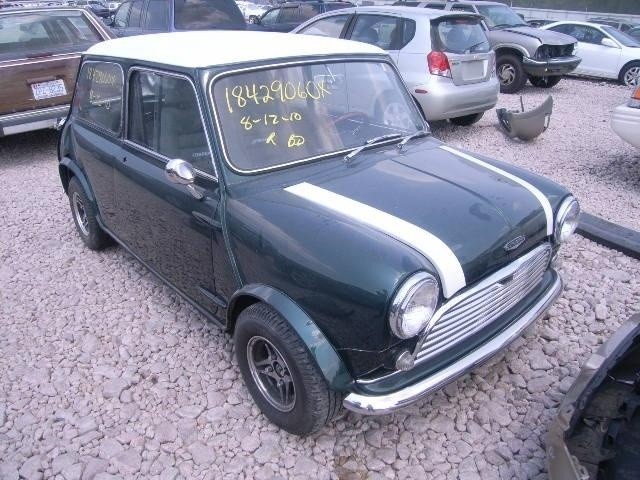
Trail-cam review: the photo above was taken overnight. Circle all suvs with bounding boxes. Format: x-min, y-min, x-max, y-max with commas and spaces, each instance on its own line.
246, 2, 357, 35
101, 0, 250, 38
391, 1, 582, 95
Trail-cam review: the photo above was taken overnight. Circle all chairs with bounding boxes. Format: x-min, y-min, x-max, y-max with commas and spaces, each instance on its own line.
108, 79, 345, 177
356, 27, 468, 51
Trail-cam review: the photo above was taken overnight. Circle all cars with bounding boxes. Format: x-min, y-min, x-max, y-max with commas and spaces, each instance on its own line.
535, 20, 640, 89
286, 7, 502, 127
518, 13, 640, 39
53, 31, 581, 438
0, 7, 128, 133
